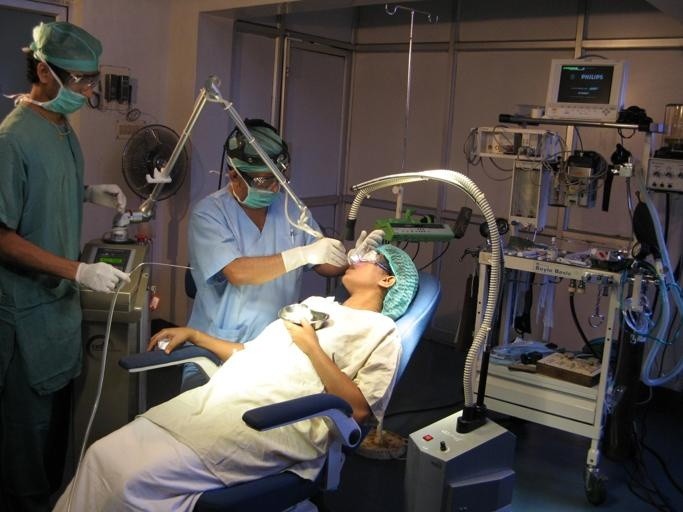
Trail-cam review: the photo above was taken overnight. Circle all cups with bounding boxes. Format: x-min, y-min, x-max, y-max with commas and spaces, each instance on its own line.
530, 108, 543, 118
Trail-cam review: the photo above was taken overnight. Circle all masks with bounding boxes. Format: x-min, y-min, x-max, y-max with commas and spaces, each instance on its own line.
232, 172, 278, 209
37, 68, 88, 114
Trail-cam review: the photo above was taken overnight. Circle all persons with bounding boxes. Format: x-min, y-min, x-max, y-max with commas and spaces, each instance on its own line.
180, 118, 385, 394
0, 21, 127, 512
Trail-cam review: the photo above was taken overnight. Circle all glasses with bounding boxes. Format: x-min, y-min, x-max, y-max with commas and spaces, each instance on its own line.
238, 168, 279, 188
52, 64, 101, 85
360, 250, 391, 274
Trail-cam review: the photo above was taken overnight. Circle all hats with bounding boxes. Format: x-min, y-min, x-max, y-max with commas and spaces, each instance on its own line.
377, 244, 420, 321
32, 22, 102, 72
227, 126, 289, 173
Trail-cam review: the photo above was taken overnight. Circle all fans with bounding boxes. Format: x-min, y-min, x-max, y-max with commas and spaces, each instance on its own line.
110, 119, 198, 423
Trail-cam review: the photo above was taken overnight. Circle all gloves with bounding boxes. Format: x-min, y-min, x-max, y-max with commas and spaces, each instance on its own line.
76, 262, 131, 294
85, 184, 127, 215
281, 230, 385, 273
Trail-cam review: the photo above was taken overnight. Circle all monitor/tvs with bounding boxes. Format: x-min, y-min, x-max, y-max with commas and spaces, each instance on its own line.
544, 58, 627, 123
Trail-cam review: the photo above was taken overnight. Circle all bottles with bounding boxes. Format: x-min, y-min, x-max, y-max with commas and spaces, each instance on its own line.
546, 234, 559, 262
